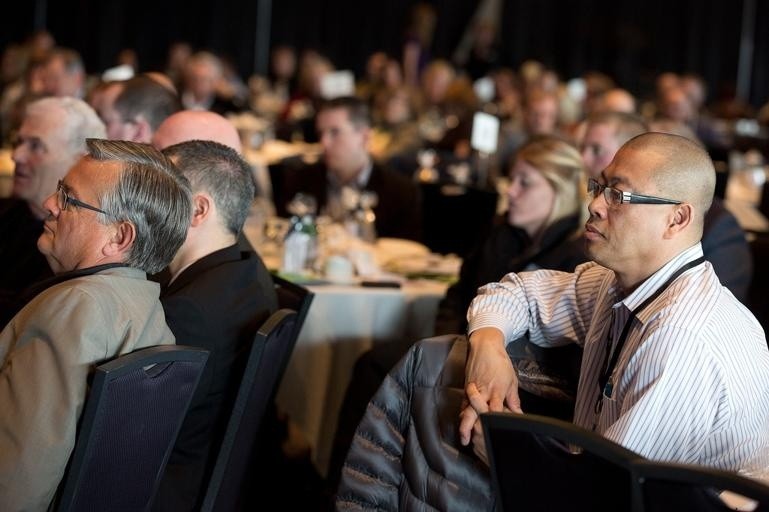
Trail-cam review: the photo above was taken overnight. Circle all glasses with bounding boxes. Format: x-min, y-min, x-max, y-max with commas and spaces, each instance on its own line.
583, 174, 686, 208
53, 178, 122, 224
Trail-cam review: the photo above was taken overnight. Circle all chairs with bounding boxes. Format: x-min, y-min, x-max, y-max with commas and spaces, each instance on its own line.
197, 304, 299, 511
475, 408, 733, 510
267, 266, 317, 331
625, 456, 767, 510
47, 343, 209, 511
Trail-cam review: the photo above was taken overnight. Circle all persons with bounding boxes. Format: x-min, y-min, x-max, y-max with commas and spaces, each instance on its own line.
0, 136, 189, 512
448, 133, 767, 511
1, 0, 769, 339
150, 137, 280, 510
